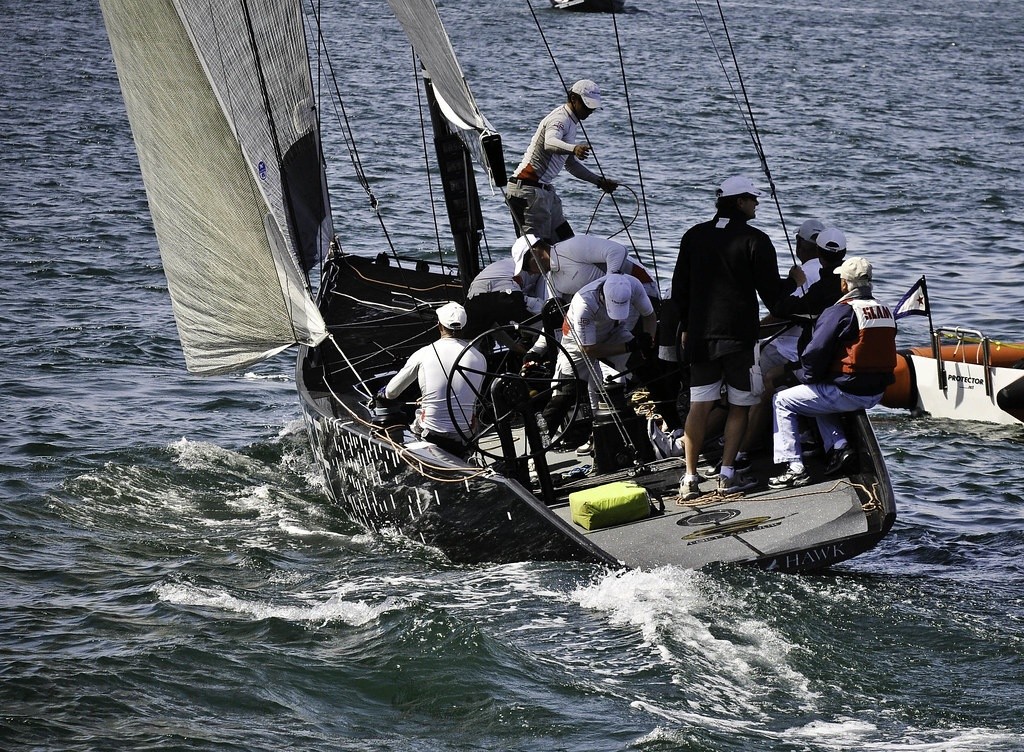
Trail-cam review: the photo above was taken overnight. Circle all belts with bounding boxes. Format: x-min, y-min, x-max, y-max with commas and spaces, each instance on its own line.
426, 428, 475, 446
507, 176, 553, 193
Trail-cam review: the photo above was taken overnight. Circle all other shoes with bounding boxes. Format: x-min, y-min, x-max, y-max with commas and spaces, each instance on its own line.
702, 435, 725, 454
576, 435, 594, 456
798, 440, 817, 457
705, 454, 752, 479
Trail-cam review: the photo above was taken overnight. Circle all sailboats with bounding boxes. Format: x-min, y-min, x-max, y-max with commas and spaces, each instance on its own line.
96, 0, 895, 595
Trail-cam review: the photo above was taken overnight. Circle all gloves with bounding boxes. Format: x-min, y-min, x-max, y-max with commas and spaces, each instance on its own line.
376, 384, 386, 400
625, 333, 653, 353
523, 351, 538, 364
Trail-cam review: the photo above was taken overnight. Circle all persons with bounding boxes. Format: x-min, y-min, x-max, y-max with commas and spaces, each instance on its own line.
511, 234, 659, 414
768, 258, 898, 490
378, 303, 487, 459
672, 176, 806, 502
508, 79, 618, 246
550, 274, 657, 456
648, 219, 847, 478
466, 257, 542, 374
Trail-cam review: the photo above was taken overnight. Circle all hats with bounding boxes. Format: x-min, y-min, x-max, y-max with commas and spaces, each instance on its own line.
569, 78, 604, 111
603, 274, 632, 321
435, 302, 467, 330
716, 176, 768, 198
816, 226, 846, 252
793, 219, 826, 244
833, 258, 872, 283
511, 234, 540, 276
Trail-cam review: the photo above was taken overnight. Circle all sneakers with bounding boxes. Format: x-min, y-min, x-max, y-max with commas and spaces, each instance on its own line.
647, 417, 684, 460
768, 464, 810, 489
678, 475, 699, 500
820, 443, 854, 474
717, 472, 760, 494
